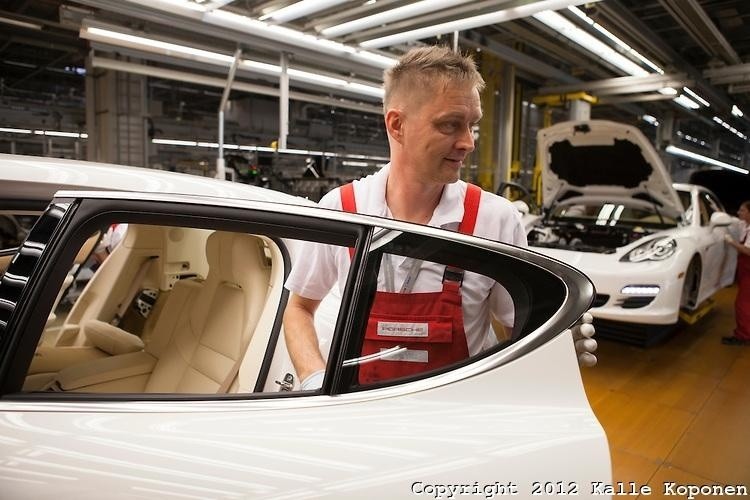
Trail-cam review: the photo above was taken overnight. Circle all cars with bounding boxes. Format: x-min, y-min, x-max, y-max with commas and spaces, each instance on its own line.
1, 153, 613, 500
525, 120, 741, 325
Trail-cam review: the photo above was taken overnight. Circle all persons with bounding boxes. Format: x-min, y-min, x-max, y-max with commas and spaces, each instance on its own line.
722, 201, 750, 345
281, 44, 598, 390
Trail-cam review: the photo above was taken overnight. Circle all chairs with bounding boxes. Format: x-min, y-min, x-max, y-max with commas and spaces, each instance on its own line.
24, 223, 268, 398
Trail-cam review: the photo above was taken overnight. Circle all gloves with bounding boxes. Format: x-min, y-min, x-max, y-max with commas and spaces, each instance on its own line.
724, 234, 734, 244
572, 311, 602, 370
301, 365, 327, 391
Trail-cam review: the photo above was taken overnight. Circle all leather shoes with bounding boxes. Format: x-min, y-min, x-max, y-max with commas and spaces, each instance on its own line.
721, 336, 750, 346
